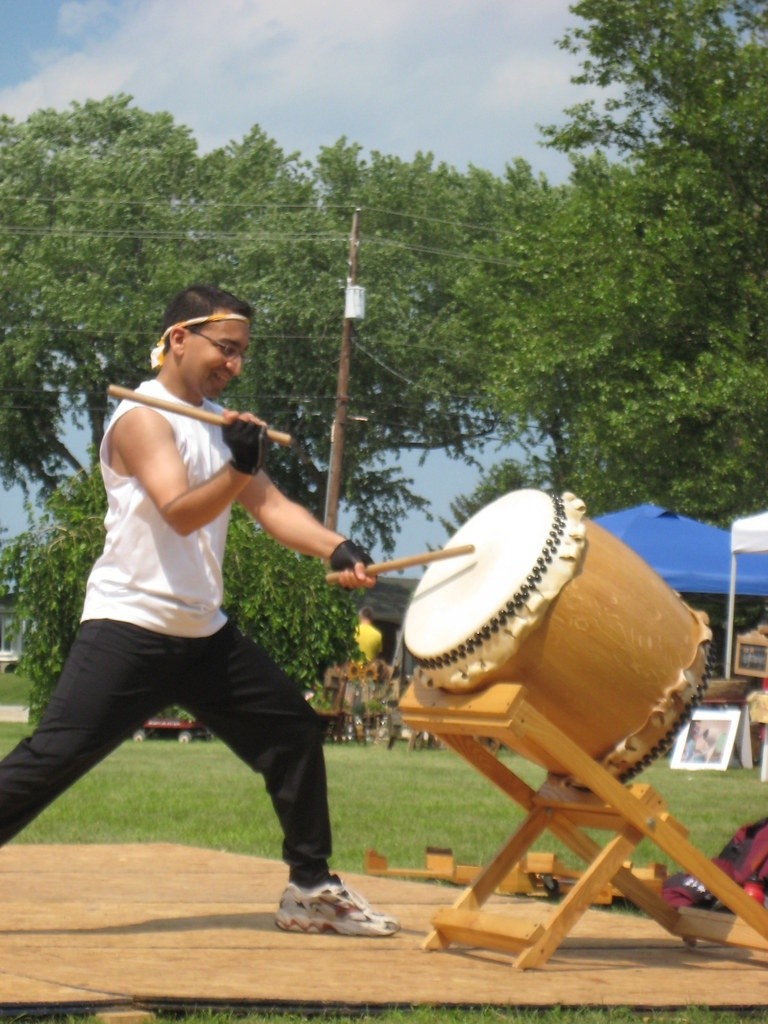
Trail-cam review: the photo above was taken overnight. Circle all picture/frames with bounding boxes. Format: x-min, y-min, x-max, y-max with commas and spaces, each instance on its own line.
733, 635, 768, 679
669, 709, 741, 772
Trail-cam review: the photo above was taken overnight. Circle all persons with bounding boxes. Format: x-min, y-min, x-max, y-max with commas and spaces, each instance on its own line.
0, 281, 406, 941
348, 606, 384, 742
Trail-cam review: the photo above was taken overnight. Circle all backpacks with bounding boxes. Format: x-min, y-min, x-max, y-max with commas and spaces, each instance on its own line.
661, 817, 768, 908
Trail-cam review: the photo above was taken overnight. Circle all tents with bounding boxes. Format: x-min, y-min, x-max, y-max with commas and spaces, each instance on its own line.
590, 500, 768, 679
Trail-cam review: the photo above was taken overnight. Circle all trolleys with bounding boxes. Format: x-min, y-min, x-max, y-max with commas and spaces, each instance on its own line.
131, 714, 209, 744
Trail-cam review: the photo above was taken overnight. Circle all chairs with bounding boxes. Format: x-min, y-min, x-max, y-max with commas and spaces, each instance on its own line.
315, 675, 349, 744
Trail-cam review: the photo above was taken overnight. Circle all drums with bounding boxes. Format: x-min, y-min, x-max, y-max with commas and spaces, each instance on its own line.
401, 488, 709, 786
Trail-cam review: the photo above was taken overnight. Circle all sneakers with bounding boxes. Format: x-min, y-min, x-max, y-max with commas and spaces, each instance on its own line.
274, 872, 402, 938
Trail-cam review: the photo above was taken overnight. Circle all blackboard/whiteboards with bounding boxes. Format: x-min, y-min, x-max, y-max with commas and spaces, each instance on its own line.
735, 635, 768, 677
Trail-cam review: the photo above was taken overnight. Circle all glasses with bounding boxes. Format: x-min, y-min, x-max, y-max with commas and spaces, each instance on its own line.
187, 326, 245, 362
